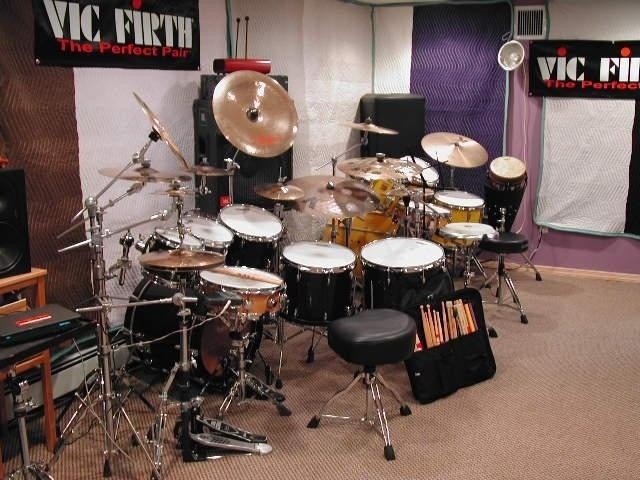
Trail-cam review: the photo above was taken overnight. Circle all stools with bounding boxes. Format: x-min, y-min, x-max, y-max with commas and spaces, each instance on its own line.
308, 308, 418, 461
479, 232, 530, 325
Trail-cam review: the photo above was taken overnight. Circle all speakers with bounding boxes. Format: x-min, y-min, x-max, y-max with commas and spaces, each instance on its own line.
0, 169, 32, 279
361, 94, 426, 159
193, 75, 293, 270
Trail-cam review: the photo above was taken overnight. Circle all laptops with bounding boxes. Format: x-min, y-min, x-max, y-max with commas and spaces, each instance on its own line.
0, 303, 82, 347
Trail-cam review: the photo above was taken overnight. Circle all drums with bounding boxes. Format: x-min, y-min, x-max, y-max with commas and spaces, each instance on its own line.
175, 214, 233, 254
216, 204, 285, 269
199, 266, 286, 322
432, 190, 484, 249
360, 237, 445, 313
145, 227, 206, 253
124, 278, 263, 383
484, 157, 528, 234
400, 155, 439, 202
394, 201, 450, 234
279, 240, 357, 325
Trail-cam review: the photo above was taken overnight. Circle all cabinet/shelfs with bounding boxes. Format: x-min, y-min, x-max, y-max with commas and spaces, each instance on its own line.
0, 266, 57, 480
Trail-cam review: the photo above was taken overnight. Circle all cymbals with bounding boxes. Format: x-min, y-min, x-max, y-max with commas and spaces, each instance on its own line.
285, 175, 380, 217
213, 70, 298, 158
337, 123, 399, 135
182, 166, 230, 176
421, 132, 489, 168
97, 168, 191, 182
254, 183, 305, 202
134, 92, 188, 168
137, 252, 224, 270
338, 157, 422, 181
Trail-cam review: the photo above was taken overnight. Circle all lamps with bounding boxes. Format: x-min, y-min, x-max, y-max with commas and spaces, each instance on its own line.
497, 40, 529, 235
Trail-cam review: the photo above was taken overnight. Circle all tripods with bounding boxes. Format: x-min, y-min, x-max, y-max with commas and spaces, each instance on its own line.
54, 142, 292, 480
8, 375, 54, 480
